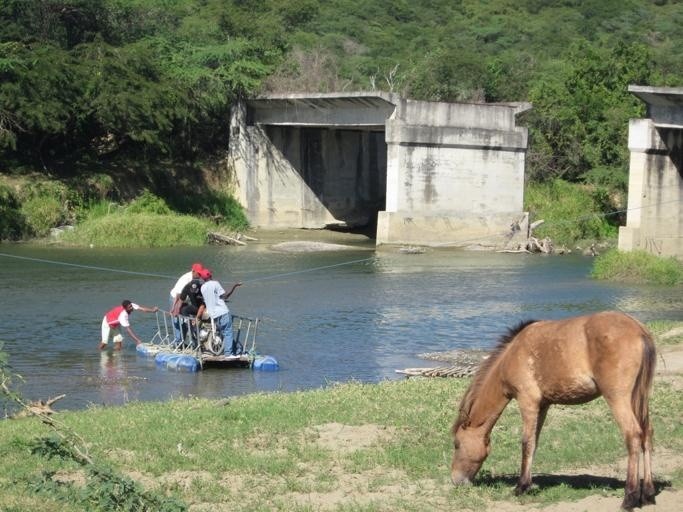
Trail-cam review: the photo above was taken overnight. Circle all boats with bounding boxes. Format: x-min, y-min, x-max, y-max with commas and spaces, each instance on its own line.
136, 310, 280, 372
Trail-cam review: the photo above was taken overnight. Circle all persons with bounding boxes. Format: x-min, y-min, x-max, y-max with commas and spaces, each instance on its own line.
173, 272, 205, 351
198, 268, 243, 359
169, 263, 203, 350
97, 299, 158, 351
100, 350, 125, 377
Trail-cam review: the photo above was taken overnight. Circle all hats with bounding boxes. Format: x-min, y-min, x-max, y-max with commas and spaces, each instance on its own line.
193, 264, 211, 279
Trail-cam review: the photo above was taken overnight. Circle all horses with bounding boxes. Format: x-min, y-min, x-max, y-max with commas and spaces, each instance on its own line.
450, 310, 658, 512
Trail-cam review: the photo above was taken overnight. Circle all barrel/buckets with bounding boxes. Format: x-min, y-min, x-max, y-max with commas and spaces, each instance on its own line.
252, 354, 280, 371
136, 342, 199, 374
